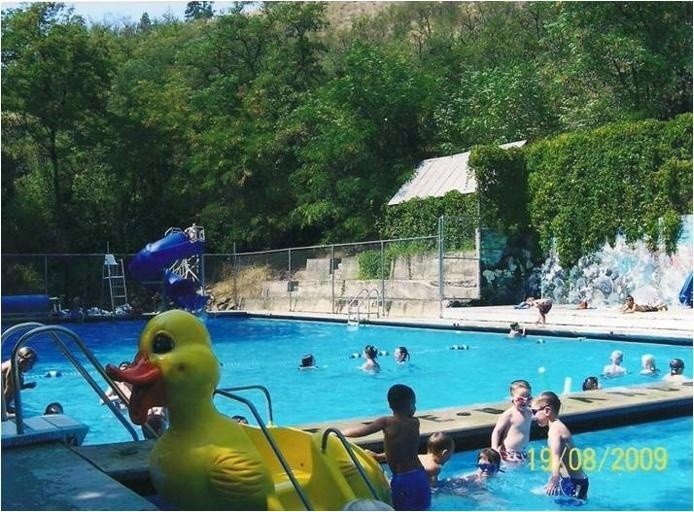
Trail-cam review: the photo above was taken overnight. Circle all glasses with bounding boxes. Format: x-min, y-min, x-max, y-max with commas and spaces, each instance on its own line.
532, 408, 544, 416
514, 396, 532, 402
477, 464, 496, 472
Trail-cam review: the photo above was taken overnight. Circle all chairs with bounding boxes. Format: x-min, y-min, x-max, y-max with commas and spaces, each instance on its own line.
104, 254, 120, 276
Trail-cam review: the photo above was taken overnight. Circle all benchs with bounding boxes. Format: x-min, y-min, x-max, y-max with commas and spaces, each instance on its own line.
332, 299, 393, 318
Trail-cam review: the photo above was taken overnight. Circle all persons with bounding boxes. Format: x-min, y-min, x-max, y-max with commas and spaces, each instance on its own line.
1, 346, 38, 413
43, 402, 63, 415
331, 379, 590, 510
99, 362, 168, 441
583, 350, 685, 391
301, 345, 410, 375
508, 296, 668, 337
234, 415, 248, 424
